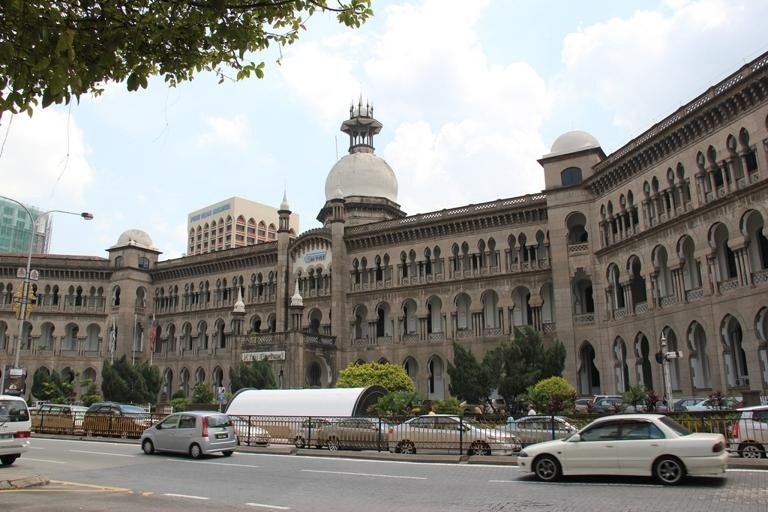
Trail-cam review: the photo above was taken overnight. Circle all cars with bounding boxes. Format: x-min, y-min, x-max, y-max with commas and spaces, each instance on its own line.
493, 415, 580, 449
592, 394, 628, 411
225, 413, 271, 448
458, 398, 507, 413
387, 413, 524, 457
315, 418, 391, 452
138, 410, 238, 460
514, 413, 730, 487
672, 397, 736, 415
413, 399, 442, 412
574, 398, 594, 413
286, 417, 343, 449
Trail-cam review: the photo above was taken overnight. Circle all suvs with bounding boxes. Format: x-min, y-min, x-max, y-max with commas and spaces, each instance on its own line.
725, 404, 767, 460
25, 398, 162, 439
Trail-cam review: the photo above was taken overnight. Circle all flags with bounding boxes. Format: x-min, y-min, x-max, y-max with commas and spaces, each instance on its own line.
106, 329, 116, 355
148, 318, 158, 353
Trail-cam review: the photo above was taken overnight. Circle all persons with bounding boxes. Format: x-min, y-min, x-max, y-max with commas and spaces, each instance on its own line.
504, 413, 516, 423
525, 403, 536, 415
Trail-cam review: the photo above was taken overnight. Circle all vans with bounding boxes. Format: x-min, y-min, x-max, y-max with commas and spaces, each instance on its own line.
0, 394, 33, 466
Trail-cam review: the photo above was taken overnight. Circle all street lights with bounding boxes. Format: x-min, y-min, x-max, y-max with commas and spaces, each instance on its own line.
0, 195, 93, 369
